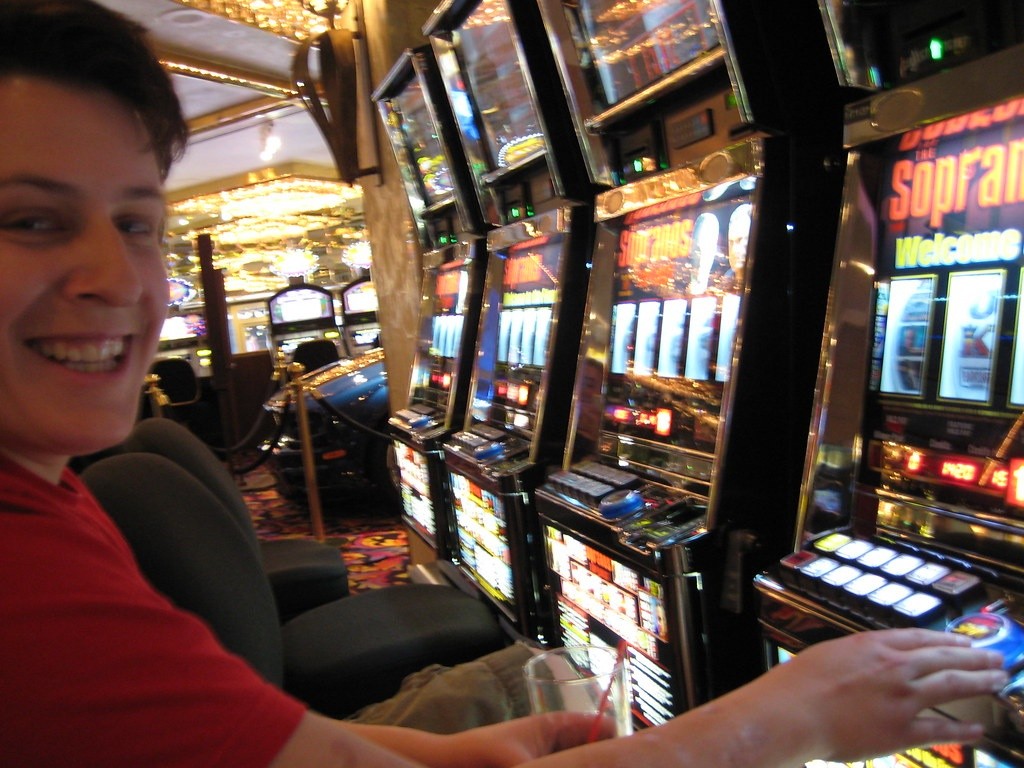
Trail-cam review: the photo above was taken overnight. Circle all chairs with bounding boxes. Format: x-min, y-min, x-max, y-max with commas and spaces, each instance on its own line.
75, 454, 504, 722
126, 416, 351, 625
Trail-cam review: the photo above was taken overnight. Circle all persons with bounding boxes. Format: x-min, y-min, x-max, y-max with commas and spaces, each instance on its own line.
1, 0, 1007, 768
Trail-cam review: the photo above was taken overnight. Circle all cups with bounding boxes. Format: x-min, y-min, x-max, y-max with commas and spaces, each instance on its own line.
521, 645, 634, 759
